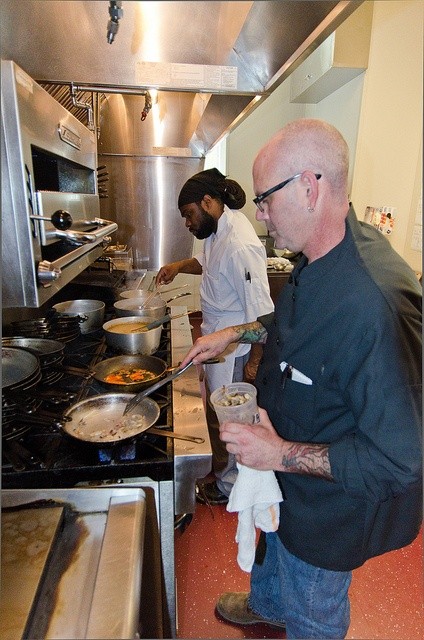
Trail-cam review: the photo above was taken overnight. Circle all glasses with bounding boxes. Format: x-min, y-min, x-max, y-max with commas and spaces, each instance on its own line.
253, 173, 321, 213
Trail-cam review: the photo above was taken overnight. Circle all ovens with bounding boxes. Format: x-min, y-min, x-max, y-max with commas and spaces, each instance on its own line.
0, 59, 117, 309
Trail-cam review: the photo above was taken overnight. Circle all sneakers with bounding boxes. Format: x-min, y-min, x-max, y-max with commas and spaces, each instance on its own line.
216, 591, 285, 629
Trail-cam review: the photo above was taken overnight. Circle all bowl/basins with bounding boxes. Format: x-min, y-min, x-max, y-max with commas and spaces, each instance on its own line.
272, 263, 288, 271
51, 300, 104, 336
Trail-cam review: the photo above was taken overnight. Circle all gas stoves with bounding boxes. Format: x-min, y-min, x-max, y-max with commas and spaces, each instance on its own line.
0, 305, 214, 516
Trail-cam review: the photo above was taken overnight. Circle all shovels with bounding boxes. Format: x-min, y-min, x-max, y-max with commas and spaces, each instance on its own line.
123, 361, 192, 416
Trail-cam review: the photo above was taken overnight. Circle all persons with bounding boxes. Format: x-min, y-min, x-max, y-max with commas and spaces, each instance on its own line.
178, 119, 424, 640
155, 168, 276, 505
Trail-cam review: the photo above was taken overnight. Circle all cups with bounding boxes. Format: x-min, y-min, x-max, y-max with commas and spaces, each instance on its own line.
209, 381, 258, 425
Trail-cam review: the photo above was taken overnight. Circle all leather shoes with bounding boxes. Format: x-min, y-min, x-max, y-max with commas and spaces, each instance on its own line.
196, 480, 228, 504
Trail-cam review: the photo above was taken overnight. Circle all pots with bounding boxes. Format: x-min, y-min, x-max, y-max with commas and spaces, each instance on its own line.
9, 313, 85, 349
113, 290, 191, 316
0, 347, 73, 442
113, 292, 122, 318
0, 307, 93, 467
102, 308, 199, 356
92, 354, 220, 393
1, 335, 96, 385
118, 278, 189, 299
60, 393, 205, 450
